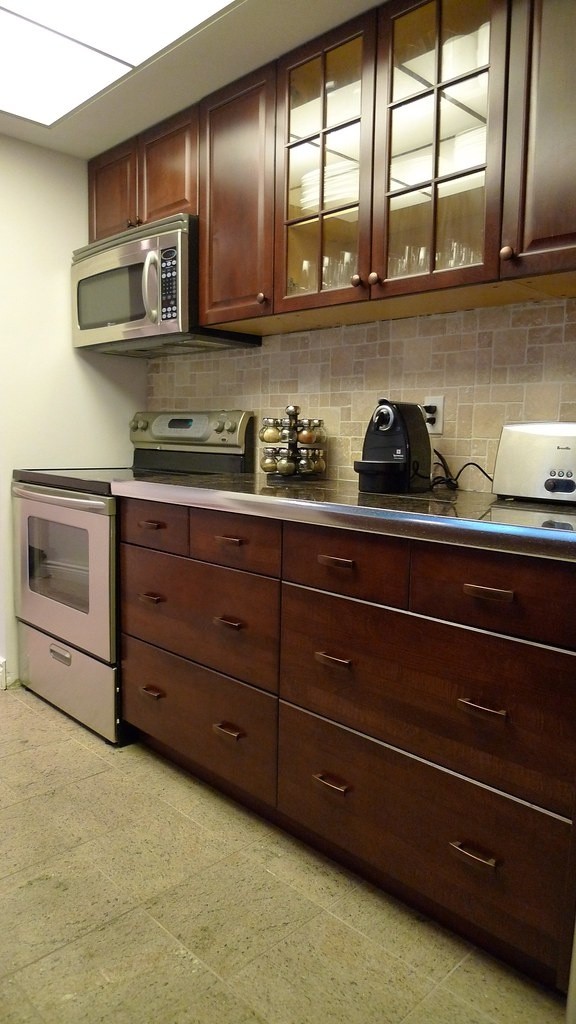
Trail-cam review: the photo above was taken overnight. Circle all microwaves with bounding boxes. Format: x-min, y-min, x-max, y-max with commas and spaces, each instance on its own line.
70, 211, 264, 360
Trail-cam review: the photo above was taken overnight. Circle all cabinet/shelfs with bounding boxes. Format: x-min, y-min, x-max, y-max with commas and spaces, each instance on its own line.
88, 0, 576, 337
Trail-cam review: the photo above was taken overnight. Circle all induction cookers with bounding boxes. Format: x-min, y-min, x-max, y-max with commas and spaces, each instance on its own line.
12, 410, 253, 495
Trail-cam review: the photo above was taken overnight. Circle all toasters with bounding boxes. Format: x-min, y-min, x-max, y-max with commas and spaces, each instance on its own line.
490, 423, 576, 505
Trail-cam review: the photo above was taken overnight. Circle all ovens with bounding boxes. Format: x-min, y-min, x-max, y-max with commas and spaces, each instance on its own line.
12, 479, 120, 744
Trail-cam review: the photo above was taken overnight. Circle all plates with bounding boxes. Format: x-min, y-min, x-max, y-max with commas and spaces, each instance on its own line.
299, 121, 485, 212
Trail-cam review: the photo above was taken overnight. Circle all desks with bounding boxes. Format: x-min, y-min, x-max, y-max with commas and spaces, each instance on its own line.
110, 472, 576, 994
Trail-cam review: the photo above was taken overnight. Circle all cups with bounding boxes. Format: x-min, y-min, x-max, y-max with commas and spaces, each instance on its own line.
299, 240, 483, 293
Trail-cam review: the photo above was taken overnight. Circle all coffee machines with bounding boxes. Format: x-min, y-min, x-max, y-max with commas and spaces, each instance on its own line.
354, 398, 432, 495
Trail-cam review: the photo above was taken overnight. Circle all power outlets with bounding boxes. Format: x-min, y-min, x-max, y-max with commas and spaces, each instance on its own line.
422, 395, 444, 435
